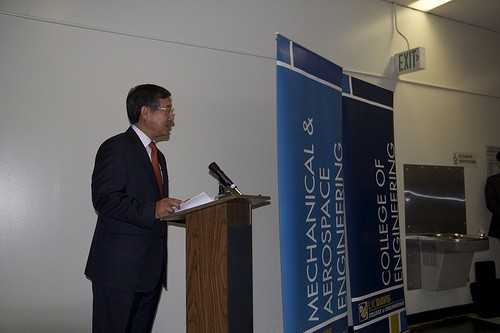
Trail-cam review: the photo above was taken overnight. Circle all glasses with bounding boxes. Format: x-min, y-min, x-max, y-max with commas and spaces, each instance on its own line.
151, 107, 176, 115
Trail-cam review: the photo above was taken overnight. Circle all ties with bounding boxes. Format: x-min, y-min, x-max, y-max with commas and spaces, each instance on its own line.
149, 142, 164, 198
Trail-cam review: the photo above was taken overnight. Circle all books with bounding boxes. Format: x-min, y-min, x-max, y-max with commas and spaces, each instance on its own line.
172, 192, 225, 213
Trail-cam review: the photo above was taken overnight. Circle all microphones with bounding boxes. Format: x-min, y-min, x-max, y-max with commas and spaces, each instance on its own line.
209, 162, 243, 195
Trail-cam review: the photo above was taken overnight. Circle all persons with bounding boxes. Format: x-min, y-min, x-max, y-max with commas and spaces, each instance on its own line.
85, 84, 183, 333
484, 151, 500, 278
